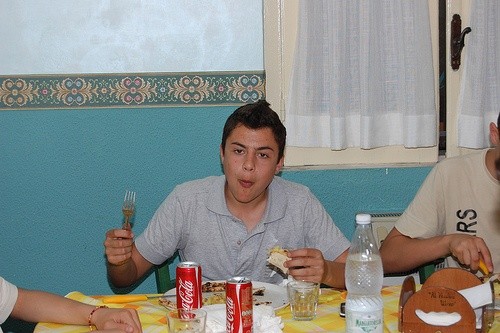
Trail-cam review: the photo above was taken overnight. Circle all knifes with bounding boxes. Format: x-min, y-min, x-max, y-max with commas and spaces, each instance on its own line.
101, 293, 177, 304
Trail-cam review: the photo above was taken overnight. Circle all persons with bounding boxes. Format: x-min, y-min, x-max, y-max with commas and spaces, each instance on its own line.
0, 276, 143, 333
378, 112, 500, 278
103, 102, 351, 295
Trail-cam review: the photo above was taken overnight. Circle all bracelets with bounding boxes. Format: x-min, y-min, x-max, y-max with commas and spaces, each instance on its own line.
89, 305, 109, 327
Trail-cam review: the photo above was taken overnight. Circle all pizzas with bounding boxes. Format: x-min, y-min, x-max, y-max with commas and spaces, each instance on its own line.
202, 282, 224, 291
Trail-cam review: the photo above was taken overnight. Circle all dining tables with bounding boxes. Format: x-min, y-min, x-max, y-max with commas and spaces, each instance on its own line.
33, 285, 500, 333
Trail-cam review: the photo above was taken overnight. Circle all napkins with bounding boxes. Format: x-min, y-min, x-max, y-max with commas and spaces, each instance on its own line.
199, 304, 284, 333
415, 280, 493, 330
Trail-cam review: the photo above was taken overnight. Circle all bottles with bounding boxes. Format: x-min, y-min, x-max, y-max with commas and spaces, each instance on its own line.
344, 213, 386, 333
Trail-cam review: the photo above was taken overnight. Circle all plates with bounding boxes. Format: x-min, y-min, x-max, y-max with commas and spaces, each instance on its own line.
161, 280, 291, 311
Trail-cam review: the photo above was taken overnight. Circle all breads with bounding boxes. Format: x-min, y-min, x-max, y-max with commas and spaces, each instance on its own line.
267, 246, 293, 275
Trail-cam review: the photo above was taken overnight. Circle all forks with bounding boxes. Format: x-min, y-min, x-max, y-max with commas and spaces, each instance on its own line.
122, 190, 136, 231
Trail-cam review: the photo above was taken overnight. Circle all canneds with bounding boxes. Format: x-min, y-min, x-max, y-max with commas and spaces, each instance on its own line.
176, 261, 203, 319
225, 276, 253, 333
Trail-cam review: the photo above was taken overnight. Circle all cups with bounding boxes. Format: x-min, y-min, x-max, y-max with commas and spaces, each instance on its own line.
167, 308, 207, 333
286, 280, 319, 321
